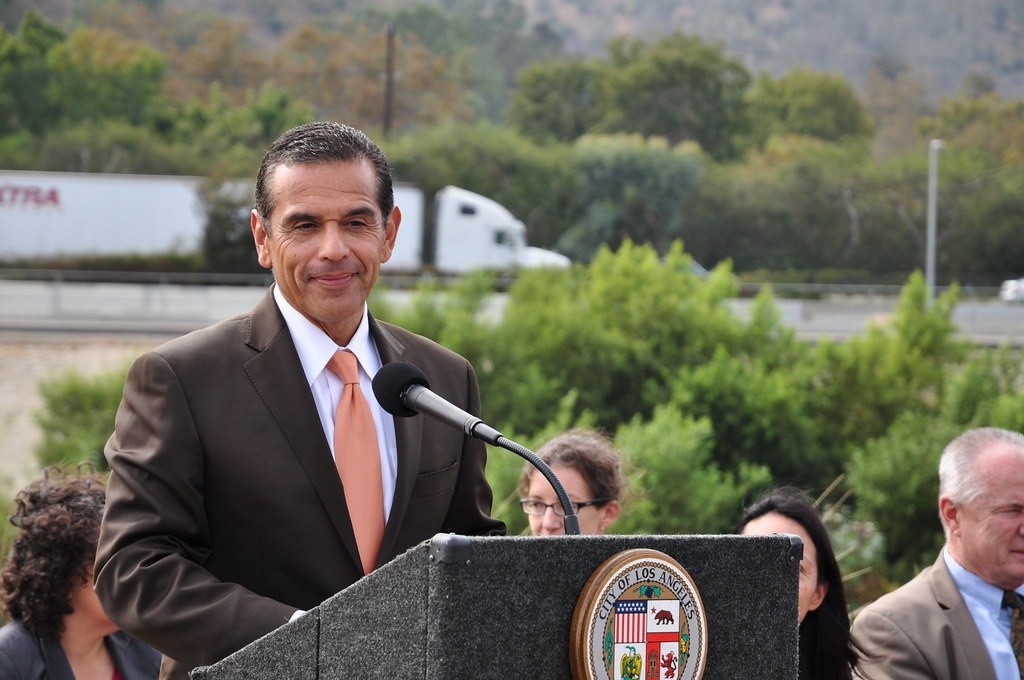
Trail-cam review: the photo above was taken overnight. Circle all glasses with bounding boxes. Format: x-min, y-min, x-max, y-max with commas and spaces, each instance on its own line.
520, 497, 611, 517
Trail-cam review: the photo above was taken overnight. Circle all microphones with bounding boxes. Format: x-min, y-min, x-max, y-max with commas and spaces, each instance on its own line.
371, 362, 503, 446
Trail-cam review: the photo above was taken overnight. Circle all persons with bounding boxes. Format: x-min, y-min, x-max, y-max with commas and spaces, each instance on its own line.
847, 425, 1023, 680
93, 121, 508, 664
515, 427, 638, 538
729, 486, 864, 680
0, 461, 168, 680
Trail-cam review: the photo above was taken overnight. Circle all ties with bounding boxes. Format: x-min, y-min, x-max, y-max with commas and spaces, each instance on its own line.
1001, 590, 1024, 680
325, 351, 386, 576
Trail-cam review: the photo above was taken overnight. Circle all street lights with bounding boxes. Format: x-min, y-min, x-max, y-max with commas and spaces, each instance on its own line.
923, 136, 949, 303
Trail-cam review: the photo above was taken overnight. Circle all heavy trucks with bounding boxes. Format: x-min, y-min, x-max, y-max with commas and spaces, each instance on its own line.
0, 169, 569, 288
